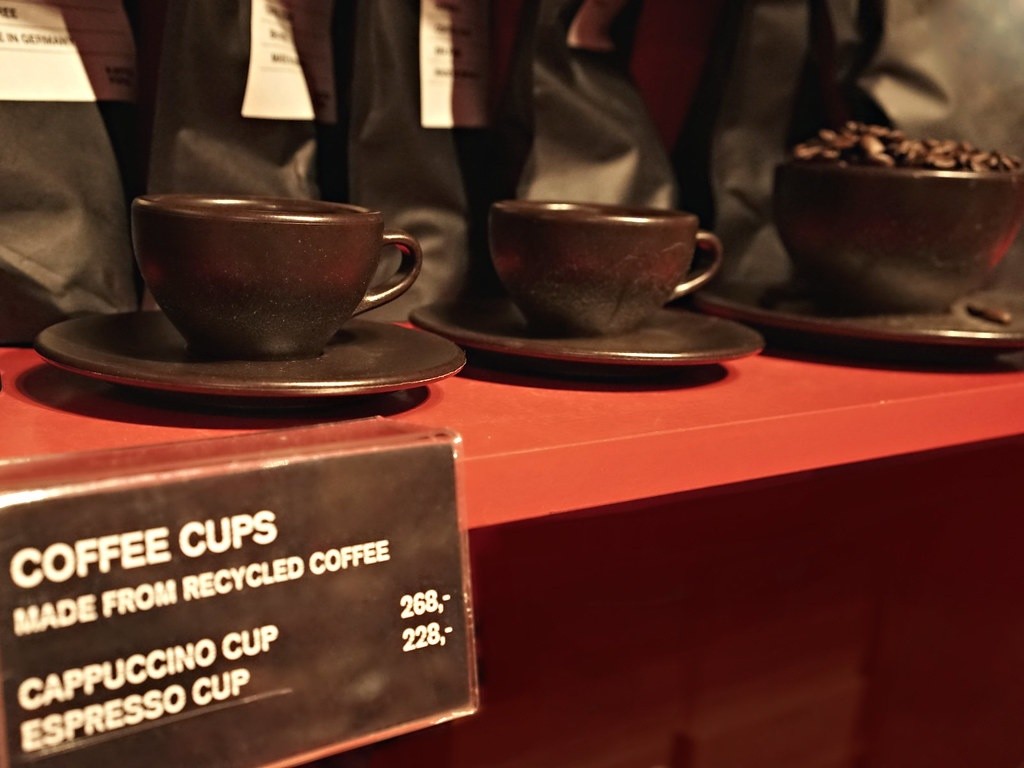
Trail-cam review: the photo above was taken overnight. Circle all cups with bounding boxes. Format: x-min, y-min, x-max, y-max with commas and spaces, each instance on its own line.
130, 190, 424, 362
770, 149, 1024, 313
489, 197, 723, 341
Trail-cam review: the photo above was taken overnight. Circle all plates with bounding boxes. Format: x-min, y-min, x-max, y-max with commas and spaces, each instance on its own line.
407, 297, 761, 370
697, 270, 1024, 360
34, 308, 466, 397
